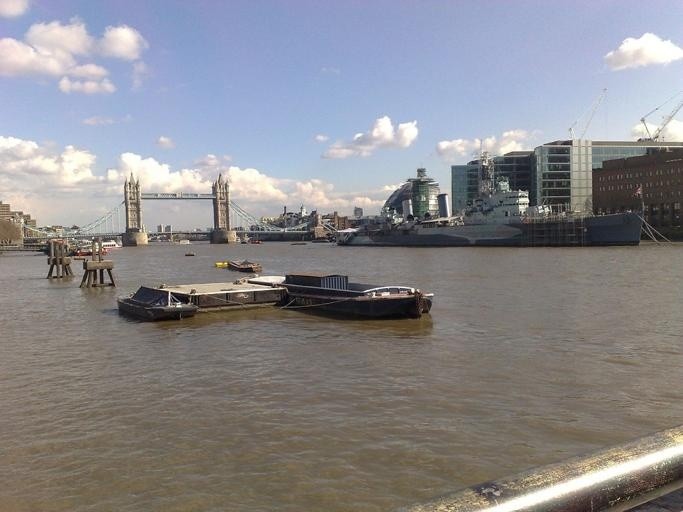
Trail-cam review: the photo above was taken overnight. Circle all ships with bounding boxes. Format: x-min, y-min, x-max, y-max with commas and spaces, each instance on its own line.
334, 151, 645, 246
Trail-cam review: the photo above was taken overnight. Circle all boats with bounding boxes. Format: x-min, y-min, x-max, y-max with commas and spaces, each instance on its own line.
116, 285, 197, 321
74, 244, 107, 256
239, 273, 432, 320
228, 260, 262, 273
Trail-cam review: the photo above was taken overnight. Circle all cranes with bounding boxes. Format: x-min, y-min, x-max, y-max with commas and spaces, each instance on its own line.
568, 88, 608, 141
639, 90, 683, 140
